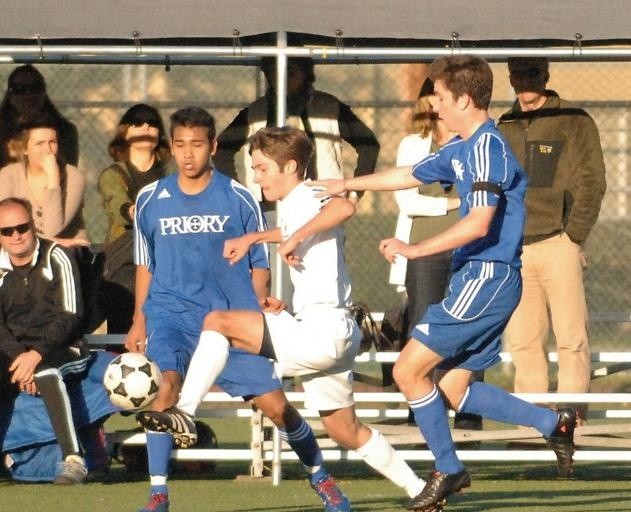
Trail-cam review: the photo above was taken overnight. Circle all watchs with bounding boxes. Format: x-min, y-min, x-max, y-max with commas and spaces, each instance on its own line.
346, 189, 360, 203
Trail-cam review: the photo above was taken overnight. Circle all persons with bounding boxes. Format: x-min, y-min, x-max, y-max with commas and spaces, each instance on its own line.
137, 126, 445, 511
211, 58, 380, 439
388, 70, 487, 450
92, 103, 178, 339
303, 53, 576, 511
0, 128, 93, 249
493, 54, 606, 426
1, 64, 77, 171
0, 198, 91, 485
123, 107, 351, 511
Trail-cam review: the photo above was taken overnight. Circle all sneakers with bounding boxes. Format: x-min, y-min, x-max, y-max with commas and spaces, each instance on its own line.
406, 468, 471, 512
311, 473, 350, 512
140, 492, 169, 512
543, 408, 579, 477
53, 459, 88, 485
136, 407, 198, 449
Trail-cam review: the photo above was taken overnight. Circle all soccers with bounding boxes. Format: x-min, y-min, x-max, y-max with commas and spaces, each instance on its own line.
104, 351, 161, 411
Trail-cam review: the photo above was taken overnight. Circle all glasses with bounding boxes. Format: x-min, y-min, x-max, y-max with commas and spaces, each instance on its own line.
10, 82, 45, 95
130, 118, 159, 127
0, 224, 28, 236
